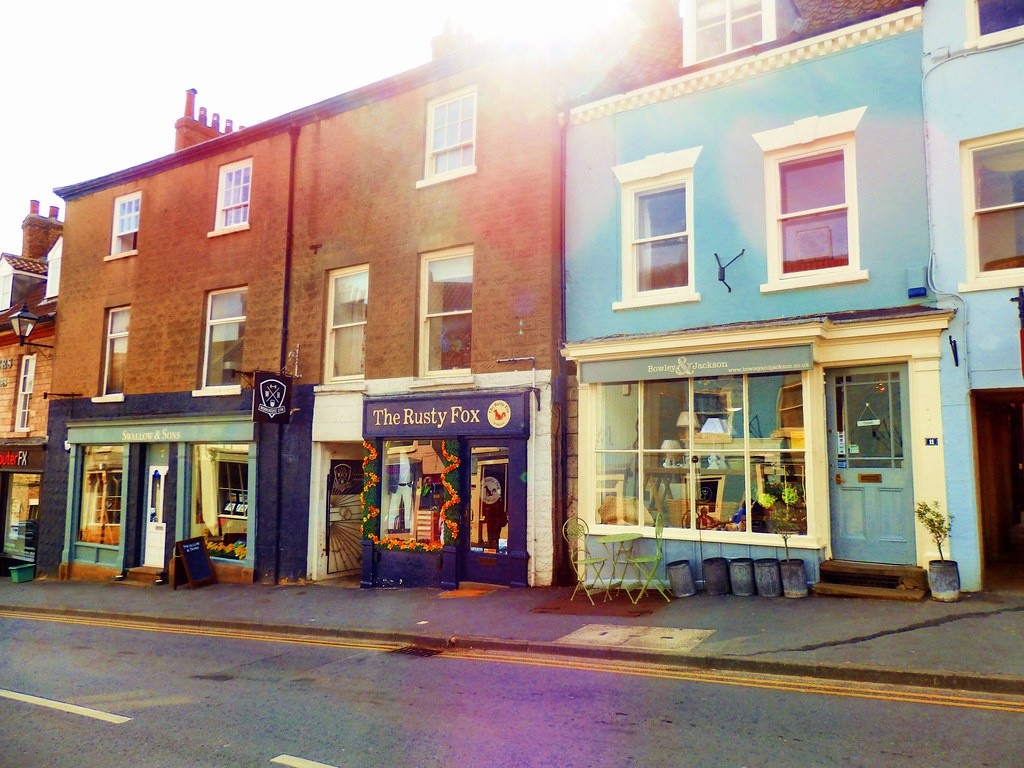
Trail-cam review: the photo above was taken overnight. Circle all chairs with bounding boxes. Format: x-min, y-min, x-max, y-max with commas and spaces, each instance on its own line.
629, 513, 673, 604
562, 516, 611, 605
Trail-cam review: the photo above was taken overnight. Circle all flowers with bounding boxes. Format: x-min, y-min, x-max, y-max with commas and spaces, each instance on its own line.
360, 439, 461, 553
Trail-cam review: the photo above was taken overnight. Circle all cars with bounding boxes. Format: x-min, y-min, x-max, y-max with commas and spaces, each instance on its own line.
8, 519, 38, 544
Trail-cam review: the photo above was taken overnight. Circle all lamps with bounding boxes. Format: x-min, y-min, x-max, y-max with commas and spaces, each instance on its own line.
676, 411, 700, 465
658, 440, 683, 468
8, 300, 54, 348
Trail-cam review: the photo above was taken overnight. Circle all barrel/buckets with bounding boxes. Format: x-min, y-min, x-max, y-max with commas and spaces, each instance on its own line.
666, 560, 697, 598
703, 557, 731, 595
753, 558, 783, 597
728, 558, 756, 597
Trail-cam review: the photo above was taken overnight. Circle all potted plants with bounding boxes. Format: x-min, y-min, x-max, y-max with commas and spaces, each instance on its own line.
758, 485, 811, 599
914, 498, 961, 601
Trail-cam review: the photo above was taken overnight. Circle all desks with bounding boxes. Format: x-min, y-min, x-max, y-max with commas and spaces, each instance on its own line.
644, 466, 791, 513
595, 533, 650, 603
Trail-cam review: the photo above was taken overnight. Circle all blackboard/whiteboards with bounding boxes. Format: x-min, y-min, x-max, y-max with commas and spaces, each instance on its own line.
173, 536, 216, 585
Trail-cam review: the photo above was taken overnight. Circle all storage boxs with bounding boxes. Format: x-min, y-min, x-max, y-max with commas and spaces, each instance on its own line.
9, 564, 36, 583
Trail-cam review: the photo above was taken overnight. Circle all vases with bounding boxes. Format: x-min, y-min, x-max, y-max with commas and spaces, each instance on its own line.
665, 556, 783, 601
